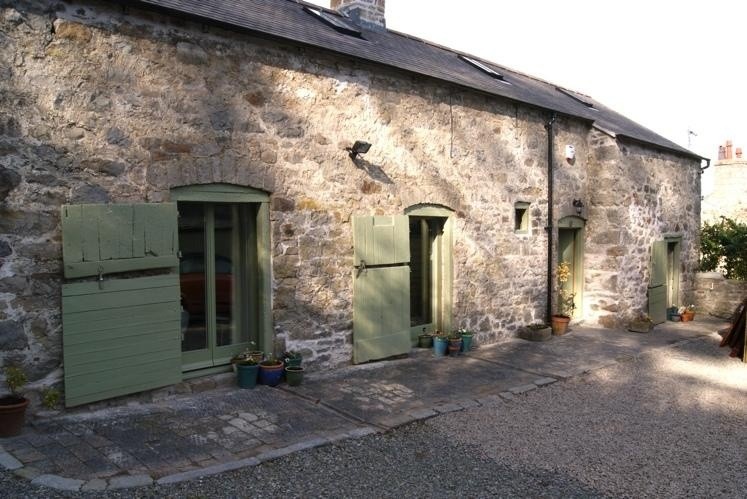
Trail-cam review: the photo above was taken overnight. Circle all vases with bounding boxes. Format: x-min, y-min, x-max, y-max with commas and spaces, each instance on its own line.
231, 350, 304, 388
416, 330, 472, 358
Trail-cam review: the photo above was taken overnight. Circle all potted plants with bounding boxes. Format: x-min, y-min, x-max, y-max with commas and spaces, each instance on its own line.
0, 363, 29, 437
670, 302, 695, 322
549, 258, 575, 335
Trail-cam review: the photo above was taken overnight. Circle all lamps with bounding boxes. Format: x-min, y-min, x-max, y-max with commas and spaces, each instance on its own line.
571, 198, 583, 216
345, 140, 372, 160
